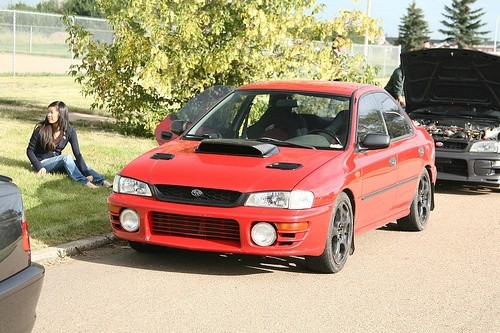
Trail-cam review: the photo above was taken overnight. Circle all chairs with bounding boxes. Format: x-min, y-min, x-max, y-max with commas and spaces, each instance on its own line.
246, 107, 359, 149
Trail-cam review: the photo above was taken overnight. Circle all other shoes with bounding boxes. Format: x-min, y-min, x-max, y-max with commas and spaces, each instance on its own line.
102, 183, 113, 188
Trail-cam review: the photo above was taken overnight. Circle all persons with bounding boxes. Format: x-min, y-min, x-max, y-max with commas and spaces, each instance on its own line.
26, 101, 113, 189
381, 65, 406, 108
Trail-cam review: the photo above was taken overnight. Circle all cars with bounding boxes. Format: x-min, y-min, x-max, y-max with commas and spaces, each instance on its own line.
0, 173, 45, 332
108, 80, 438, 273
383, 46, 500, 193
